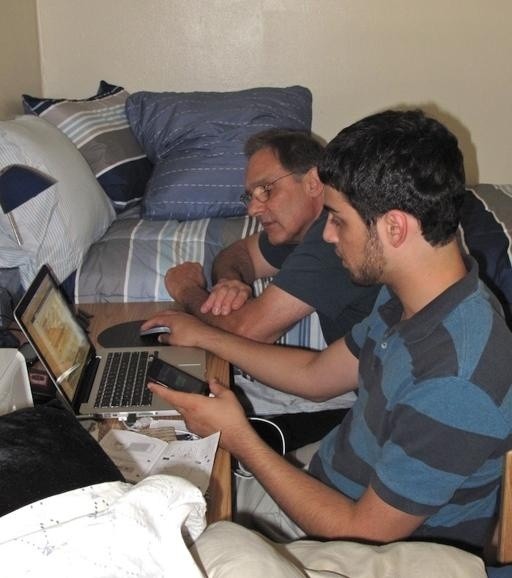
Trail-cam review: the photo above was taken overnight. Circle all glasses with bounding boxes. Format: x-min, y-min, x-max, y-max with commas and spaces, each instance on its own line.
239, 167, 308, 210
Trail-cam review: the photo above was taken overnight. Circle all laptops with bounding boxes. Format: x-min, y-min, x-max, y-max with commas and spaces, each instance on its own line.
13, 265, 208, 422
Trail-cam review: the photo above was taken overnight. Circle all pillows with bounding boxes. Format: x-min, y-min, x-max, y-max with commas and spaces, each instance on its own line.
1, 113, 120, 288
125, 82, 315, 219
21, 74, 158, 212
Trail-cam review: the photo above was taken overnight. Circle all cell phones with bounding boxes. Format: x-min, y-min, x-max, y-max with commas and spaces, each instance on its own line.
147, 359, 205, 399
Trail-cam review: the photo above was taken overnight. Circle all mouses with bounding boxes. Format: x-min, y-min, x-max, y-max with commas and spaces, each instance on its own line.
136, 325, 171, 342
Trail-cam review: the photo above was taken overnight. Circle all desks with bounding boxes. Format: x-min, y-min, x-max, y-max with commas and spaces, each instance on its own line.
0, 302, 240, 531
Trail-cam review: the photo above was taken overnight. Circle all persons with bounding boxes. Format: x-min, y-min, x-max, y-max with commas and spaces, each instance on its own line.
141, 108, 512, 556
162, 126, 382, 347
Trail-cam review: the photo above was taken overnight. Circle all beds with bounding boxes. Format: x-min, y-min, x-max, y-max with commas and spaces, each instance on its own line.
52, 180, 512, 414
